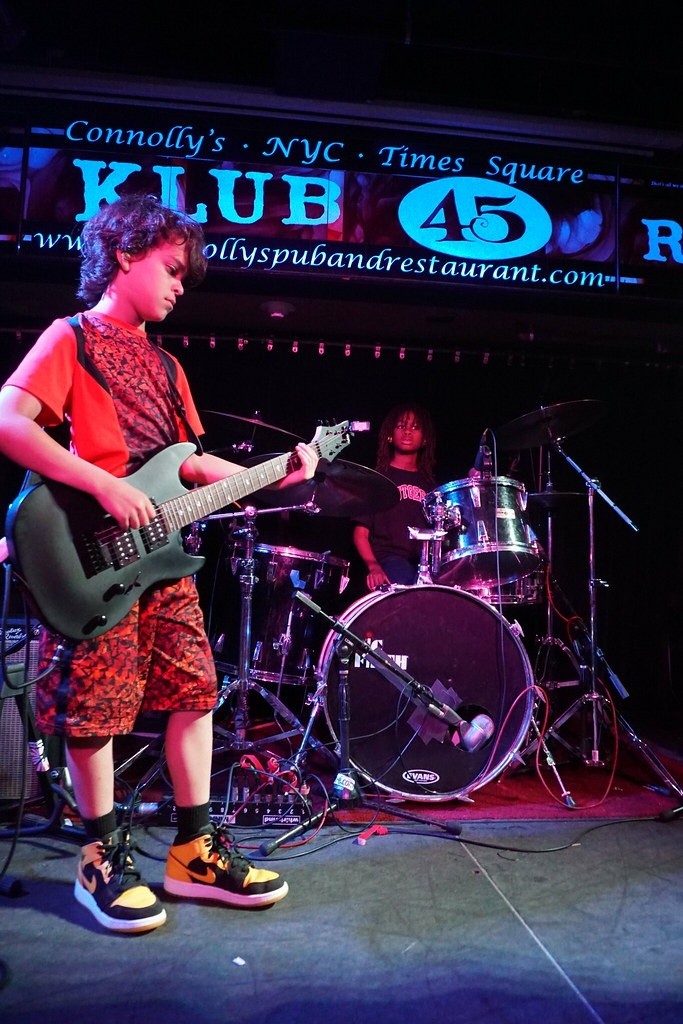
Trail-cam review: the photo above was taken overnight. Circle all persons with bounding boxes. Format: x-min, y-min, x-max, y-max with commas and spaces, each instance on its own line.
354, 401, 442, 592
0, 196, 320, 932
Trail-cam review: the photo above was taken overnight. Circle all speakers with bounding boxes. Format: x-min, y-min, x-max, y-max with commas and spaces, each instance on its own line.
0, 616, 49, 810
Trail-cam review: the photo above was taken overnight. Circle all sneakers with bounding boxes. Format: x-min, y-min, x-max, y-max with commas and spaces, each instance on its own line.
73, 826, 167, 933
164, 822, 290, 906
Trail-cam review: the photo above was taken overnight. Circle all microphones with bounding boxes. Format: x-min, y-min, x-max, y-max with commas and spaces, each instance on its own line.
462, 714, 494, 753
473, 434, 487, 471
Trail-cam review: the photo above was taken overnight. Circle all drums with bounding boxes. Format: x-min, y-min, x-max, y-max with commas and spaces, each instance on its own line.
422, 474, 544, 590
310, 580, 536, 804
211, 539, 351, 686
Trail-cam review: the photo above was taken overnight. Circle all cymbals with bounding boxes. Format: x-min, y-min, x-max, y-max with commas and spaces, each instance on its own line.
526, 490, 586, 500
488, 397, 603, 452
244, 451, 401, 518
206, 409, 302, 441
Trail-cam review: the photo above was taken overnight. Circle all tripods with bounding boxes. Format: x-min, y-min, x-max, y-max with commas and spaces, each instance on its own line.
114, 408, 683, 857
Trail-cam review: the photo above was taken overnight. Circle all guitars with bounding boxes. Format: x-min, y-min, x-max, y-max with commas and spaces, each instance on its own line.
4, 420, 357, 643
